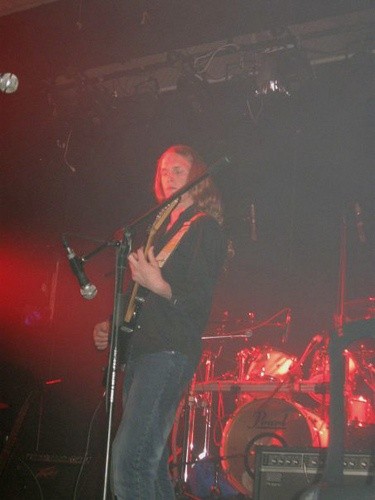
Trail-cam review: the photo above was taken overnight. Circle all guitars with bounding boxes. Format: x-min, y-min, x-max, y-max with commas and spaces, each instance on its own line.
117, 194, 181, 361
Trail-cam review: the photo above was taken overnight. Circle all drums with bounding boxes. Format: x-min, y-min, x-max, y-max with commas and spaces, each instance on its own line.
344, 349, 375, 431
236, 347, 299, 400
306, 345, 331, 404
219, 396, 329, 495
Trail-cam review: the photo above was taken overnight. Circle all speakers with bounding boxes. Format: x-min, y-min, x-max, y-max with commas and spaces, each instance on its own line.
255, 445, 375, 500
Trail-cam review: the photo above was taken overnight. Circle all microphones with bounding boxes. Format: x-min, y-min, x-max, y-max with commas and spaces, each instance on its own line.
0, 72, 19, 93
282, 309, 292, 343
62, 239, 97, 299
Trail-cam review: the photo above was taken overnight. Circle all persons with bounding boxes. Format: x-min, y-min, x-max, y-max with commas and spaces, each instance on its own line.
92, 144, 236, 500
